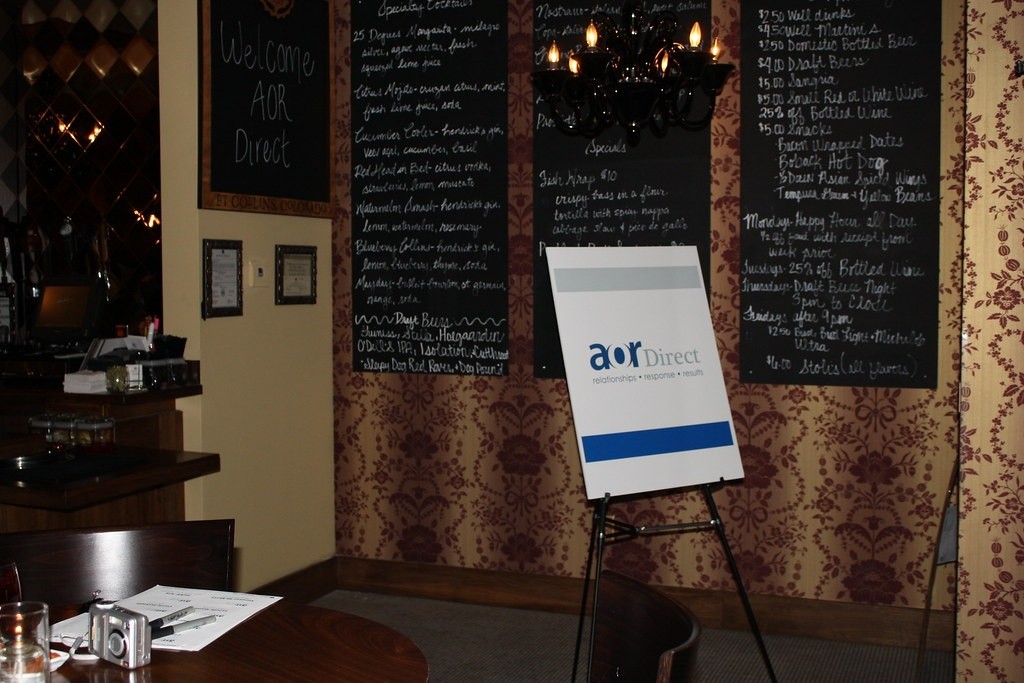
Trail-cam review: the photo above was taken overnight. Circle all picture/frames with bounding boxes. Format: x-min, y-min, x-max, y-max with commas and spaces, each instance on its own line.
275, 243, 318, 305
199, 238, 244, 321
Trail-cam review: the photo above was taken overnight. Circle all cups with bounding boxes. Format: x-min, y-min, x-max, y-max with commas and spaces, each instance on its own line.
0, 602, 51, 683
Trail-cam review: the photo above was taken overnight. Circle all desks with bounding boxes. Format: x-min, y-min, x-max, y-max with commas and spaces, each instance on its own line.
0, 597, 429, 683
0, 378, 221, 533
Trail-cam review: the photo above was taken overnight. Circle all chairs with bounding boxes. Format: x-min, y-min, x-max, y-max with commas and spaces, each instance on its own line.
588, 568, 701, 683
0, 518, 236, 607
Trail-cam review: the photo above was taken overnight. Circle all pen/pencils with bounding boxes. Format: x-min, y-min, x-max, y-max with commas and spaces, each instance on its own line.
144, 315, 159, 344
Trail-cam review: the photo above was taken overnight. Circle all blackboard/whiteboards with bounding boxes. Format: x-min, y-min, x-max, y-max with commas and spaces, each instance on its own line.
530, 1, 716, 383
198, 1, 339, 222
345, 0, 510, 380
738, 0, 942, 391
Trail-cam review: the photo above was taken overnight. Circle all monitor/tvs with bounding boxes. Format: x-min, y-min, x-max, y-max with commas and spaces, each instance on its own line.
29, 276, 104, 342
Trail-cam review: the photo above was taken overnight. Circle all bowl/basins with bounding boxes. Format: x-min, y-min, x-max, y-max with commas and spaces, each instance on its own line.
49, 649, 70, 672
72, 654, 100, 664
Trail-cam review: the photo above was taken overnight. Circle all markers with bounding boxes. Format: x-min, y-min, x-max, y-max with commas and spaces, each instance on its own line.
148, 605, 195, 628
151, 615, 216, 640
